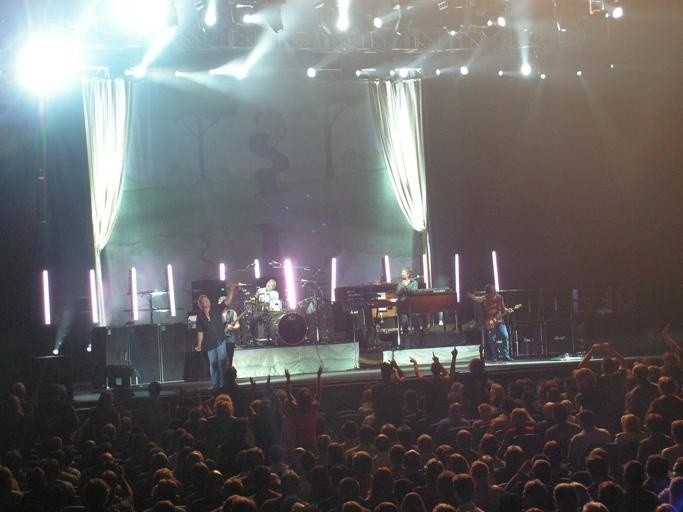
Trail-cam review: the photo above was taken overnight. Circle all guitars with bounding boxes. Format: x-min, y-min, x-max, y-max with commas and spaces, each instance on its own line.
223, 305, 252, 338
482, 303, 523, 332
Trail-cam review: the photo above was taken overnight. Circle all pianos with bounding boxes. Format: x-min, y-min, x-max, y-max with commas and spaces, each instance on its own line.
399, 287, 459, 316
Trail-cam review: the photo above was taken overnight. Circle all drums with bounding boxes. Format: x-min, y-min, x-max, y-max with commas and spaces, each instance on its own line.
250, 313, 271, 342
268, 312, 308, 346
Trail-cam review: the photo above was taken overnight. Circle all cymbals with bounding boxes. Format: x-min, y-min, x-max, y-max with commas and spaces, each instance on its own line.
231, 281, 253, 286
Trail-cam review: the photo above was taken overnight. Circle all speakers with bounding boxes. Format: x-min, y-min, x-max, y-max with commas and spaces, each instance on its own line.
159, 323, 192, 383
191, 279, 228, 313
91, 325, 129, 385
129, 323, 161, 385
191, 328, 211, 382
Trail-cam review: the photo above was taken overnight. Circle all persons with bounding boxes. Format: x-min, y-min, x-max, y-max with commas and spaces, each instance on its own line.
466, 283, 514, 363
0, 321, 682, 512
217, 295, 241, 369
193, 282, 235, 391
395, 266, 420, 335
250, 279, 279, 340
